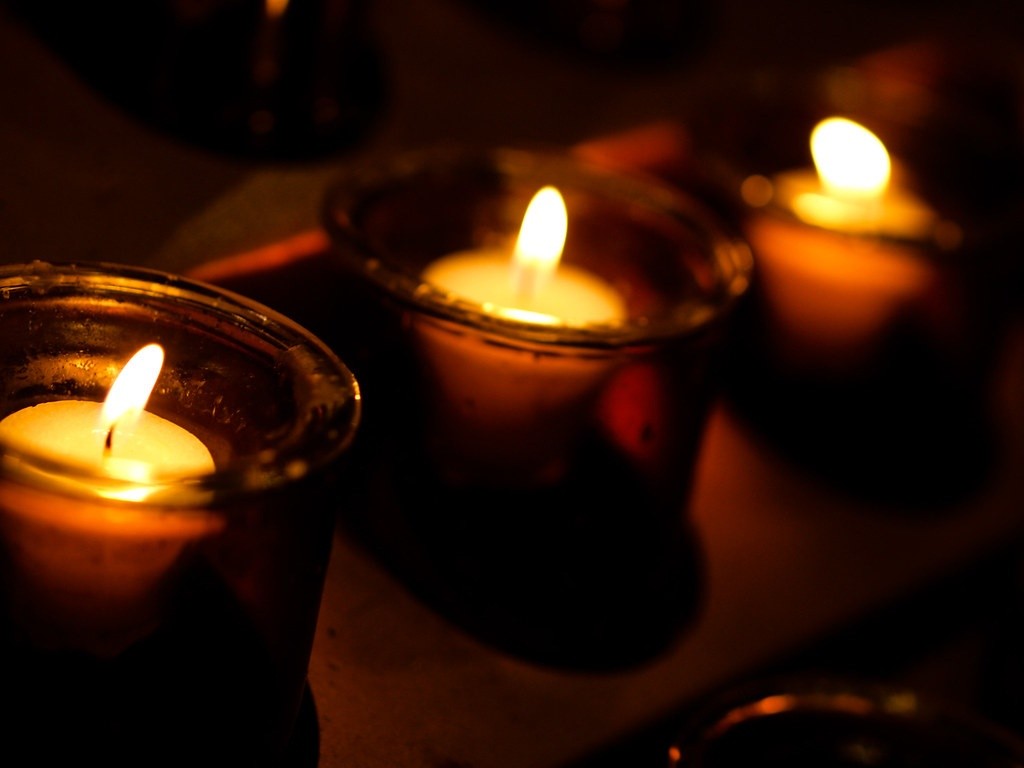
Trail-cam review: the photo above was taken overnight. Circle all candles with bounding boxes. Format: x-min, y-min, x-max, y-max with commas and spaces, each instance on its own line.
0, 2, 1024, 766
